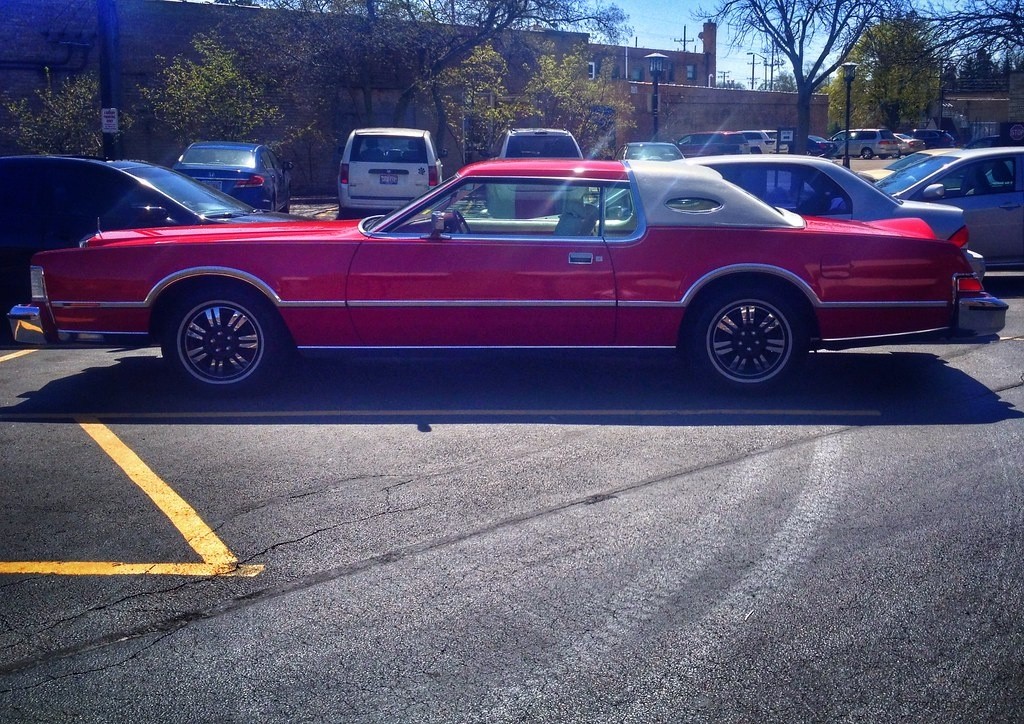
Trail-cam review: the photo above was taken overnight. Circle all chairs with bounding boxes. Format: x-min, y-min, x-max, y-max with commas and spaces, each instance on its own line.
554, 203, 599, 236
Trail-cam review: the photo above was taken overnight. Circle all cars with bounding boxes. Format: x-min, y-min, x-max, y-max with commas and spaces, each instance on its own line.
0, 154, 329, 318
7, 158, 1009, 411
172, 139, 294, 215
533, 147, 1024, 277
826, 128, 955, 160
788, 134, 837, 157
598, 142, 685, 202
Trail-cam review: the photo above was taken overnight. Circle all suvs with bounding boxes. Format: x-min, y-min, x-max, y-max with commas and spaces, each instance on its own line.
336, 127, 447, 217
475, 126, 585, 202
673, 129, 789, 159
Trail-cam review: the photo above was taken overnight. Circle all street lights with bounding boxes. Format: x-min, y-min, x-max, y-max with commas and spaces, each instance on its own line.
643, 51, 670, 141
747, 52, 767, 90
840, 58, 858, 169
